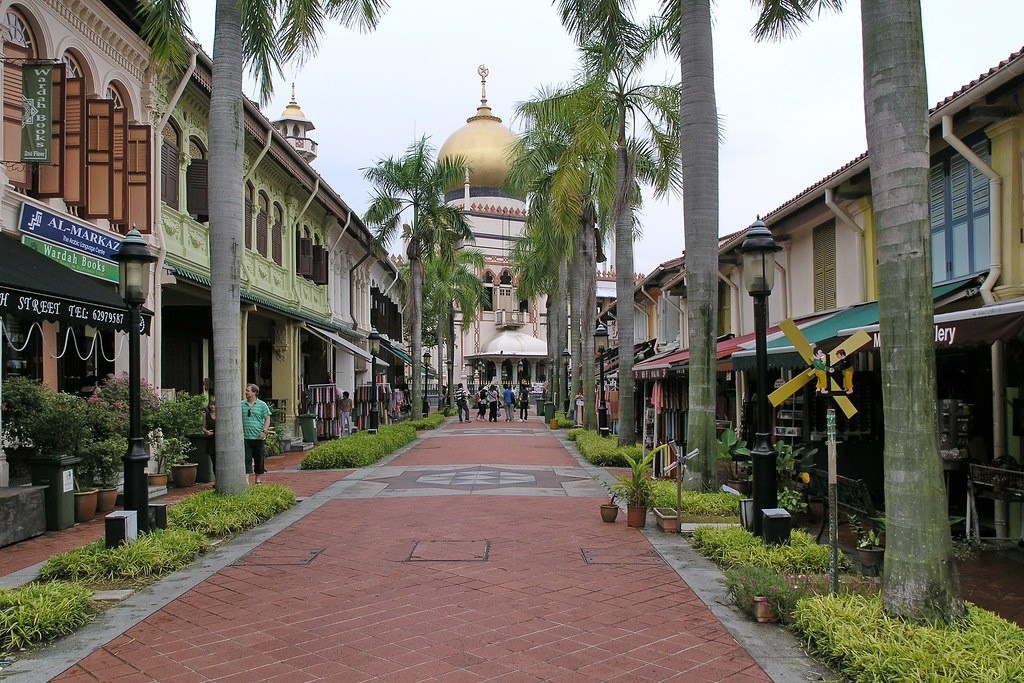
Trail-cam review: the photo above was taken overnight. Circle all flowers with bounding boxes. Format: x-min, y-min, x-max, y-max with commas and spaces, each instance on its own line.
86, 370, 161, 412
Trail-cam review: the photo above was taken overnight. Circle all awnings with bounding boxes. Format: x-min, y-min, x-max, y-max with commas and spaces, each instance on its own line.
731, 279, 973, 371
304, 325, 389, 366
382, 342, 436, 375
594, 346, 645, 378
0, 231, 155, 337
631, 313, 837, 380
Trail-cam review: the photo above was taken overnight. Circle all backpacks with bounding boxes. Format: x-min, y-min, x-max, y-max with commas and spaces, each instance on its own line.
475, 390, 483, 402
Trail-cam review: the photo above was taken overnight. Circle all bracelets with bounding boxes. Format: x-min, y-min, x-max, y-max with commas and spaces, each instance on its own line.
262, 429, 267, 434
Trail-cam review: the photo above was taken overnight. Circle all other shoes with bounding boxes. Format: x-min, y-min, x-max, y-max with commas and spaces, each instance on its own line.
459, 421, 464, 423
255, 480, 260, 486
523, 418, 527, 422
465, 420, 471, 423
475, 417, 513, 422
517, 418, 523, 422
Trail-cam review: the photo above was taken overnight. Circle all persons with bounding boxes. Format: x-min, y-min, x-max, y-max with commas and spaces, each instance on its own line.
338, 392, 352, 436
501, 384, 514, 422
475, 384, 500, 422
443, 385, 448, 405
241, 384, 272, 485
202, 397, 216, 488
510, 384, 529, 422
456, 383, 472, 423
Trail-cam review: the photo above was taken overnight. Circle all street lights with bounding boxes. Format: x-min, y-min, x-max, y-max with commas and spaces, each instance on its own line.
592, 324, 610, 435
366, 325, 382, 433
111, 223, 159, 530
422, 351, 432, 412
518, 360, 523, 395
446, 359, 452, 405
561, 348, 571, 415
735, 214, 783, 537
478, 361, 485, 391
550, 358, 554, 402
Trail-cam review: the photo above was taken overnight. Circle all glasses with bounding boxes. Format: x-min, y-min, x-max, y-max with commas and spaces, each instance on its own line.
247, 409, 251, 416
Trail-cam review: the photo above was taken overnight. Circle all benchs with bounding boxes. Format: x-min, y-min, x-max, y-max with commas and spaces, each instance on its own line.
812, 467, 886, 545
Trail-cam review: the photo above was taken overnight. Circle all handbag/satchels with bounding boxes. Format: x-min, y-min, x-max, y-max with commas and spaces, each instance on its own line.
497, 407, 501, 417
510, 392, 515, 404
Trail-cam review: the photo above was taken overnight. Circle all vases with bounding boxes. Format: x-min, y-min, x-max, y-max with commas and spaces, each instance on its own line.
753, 594, 780, 623
653, 507, 681, 532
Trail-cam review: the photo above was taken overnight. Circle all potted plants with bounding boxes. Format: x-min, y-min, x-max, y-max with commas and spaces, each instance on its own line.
592, 443, 668, 528
846, 513, 885, 565
715, 427, 819, 528
1, 376, 208, 523
